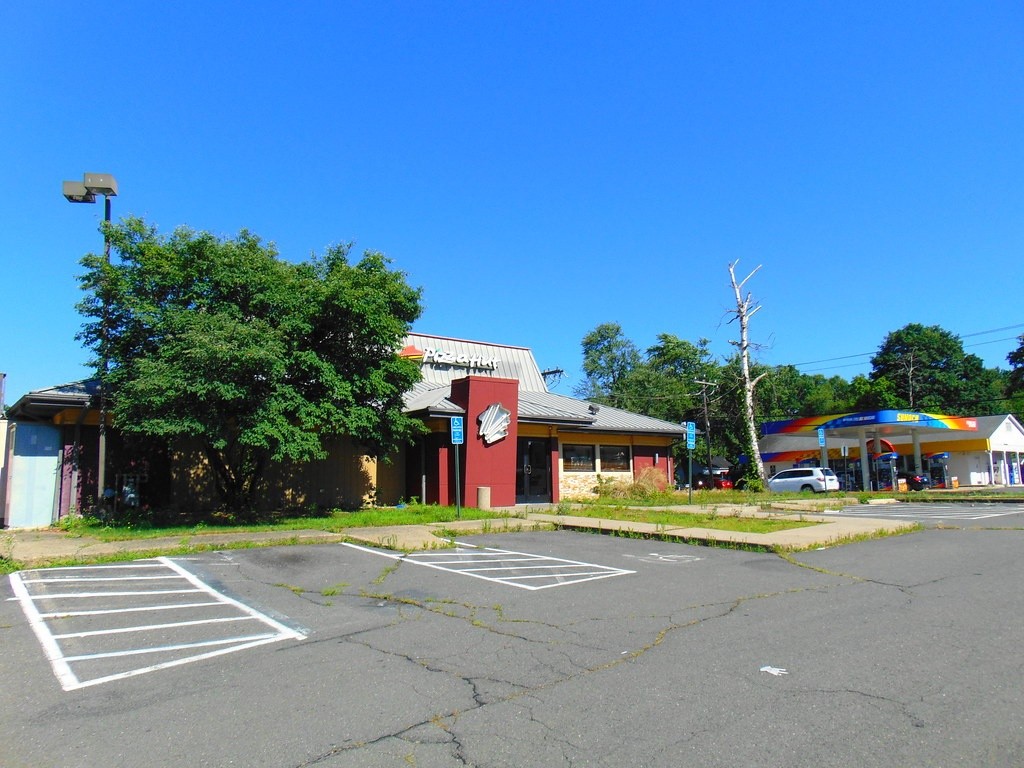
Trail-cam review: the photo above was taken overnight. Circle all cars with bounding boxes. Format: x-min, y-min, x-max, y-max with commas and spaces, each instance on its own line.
766, 466, 839, 493
898, 470, 928, 492
693, 474, 734, 490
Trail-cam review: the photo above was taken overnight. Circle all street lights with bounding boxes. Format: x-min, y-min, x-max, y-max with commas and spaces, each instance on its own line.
61, 172, 118, 517
